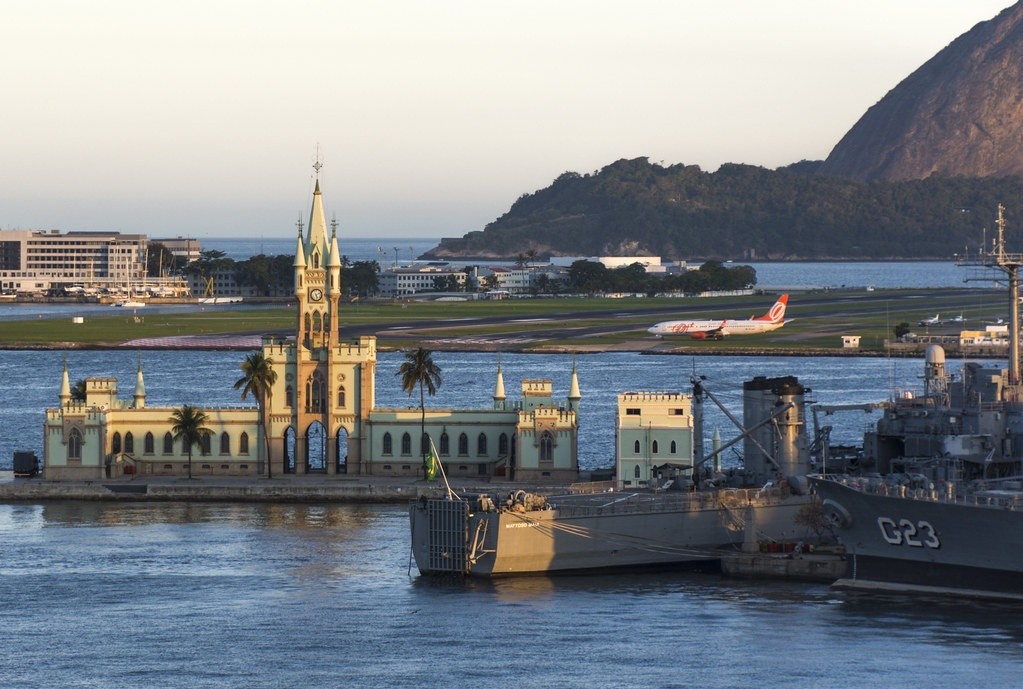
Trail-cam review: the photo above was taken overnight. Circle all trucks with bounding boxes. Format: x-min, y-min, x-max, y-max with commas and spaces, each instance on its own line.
13, 451, 41, 478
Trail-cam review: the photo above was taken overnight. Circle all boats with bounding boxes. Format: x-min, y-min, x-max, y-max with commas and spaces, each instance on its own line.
806, 442, 1023, 600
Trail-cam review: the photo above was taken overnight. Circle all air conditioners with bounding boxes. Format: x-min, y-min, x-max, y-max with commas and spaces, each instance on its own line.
888, 413, 896, 419
912, 410, 920, 417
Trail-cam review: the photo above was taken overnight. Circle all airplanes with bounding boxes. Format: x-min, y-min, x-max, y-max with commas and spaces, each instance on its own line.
647, 294, 795, 341
918, 313, 942, 326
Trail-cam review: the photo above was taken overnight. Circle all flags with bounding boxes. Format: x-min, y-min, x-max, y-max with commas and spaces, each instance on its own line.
426, 439, 438, 481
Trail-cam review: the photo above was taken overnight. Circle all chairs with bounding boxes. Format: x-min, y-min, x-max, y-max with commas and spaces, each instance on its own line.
652, 480, 674, 494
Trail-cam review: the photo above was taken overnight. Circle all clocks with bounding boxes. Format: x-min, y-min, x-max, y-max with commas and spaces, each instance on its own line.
310, 289, 322, 301
